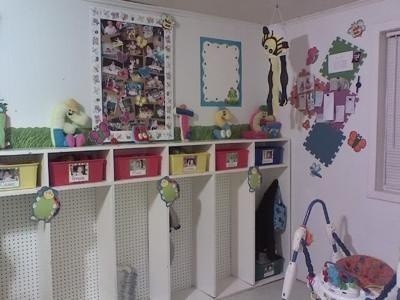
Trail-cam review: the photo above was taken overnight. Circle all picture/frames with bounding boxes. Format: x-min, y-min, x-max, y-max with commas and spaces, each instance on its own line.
89, 6, 175, 142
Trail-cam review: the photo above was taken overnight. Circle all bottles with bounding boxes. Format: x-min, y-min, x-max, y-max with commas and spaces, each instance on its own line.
0, 98, 7, 149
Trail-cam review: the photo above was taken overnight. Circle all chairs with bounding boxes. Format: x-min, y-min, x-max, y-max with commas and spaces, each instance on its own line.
300, 199, 398, 300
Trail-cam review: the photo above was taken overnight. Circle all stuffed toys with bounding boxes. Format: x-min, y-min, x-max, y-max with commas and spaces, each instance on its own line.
239, 103, 275, 139
61, 96, 90, 148
175, 104, 195, 143
210, 108, 234, 140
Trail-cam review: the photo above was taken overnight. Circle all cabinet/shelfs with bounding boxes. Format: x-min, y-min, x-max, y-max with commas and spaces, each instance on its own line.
0, 137, 292, 300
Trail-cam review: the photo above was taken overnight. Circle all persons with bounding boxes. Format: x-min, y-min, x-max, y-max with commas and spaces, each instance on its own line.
130, 159, 146, 175
69, 163, 89, 182
100, 19, 165, 131
0, 169, 19, 188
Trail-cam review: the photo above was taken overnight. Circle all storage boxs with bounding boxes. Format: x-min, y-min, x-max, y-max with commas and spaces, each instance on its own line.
115, 152, 159, 180
216, 148, 249, 171
256, 255, 283, 281
255, 147, 282, 166
169, 151, 209, 175
0, 160, 40, 192
48, 158, 105, 186
